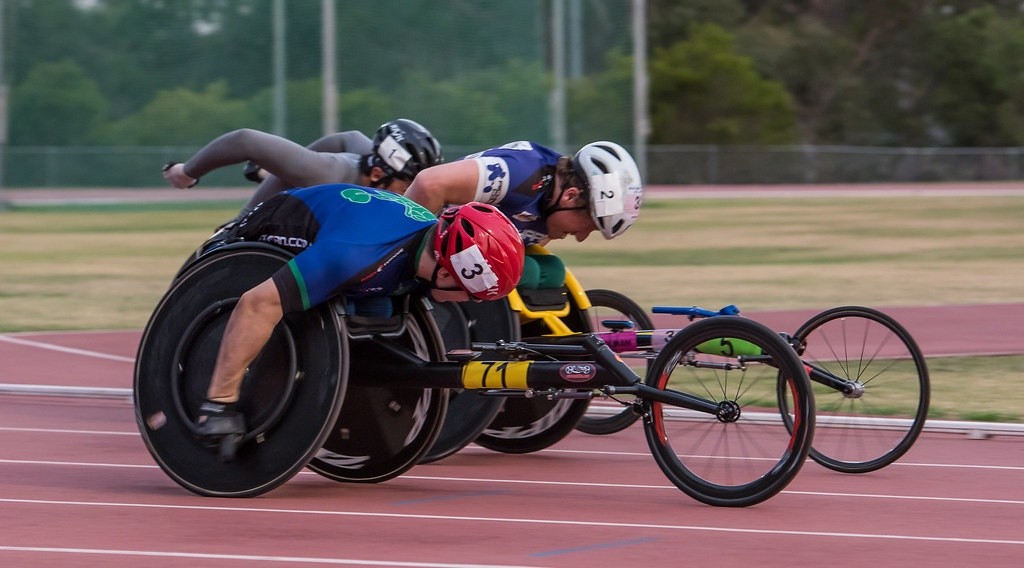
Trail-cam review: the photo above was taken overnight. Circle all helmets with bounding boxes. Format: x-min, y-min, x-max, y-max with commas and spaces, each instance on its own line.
372, 118, 444, 182
572, 141, 643, 240
433, 202, 525, 303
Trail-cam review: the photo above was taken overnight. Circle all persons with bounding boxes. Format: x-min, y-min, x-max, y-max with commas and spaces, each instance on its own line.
403, 139, 642, 291
161, 114, 442, 220
197, 182, 525, 462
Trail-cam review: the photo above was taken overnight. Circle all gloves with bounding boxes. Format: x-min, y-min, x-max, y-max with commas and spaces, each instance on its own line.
192, 398, 247, 463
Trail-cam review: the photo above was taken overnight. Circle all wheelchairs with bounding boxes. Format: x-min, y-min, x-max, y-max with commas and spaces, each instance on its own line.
132, 218, 931, 498
